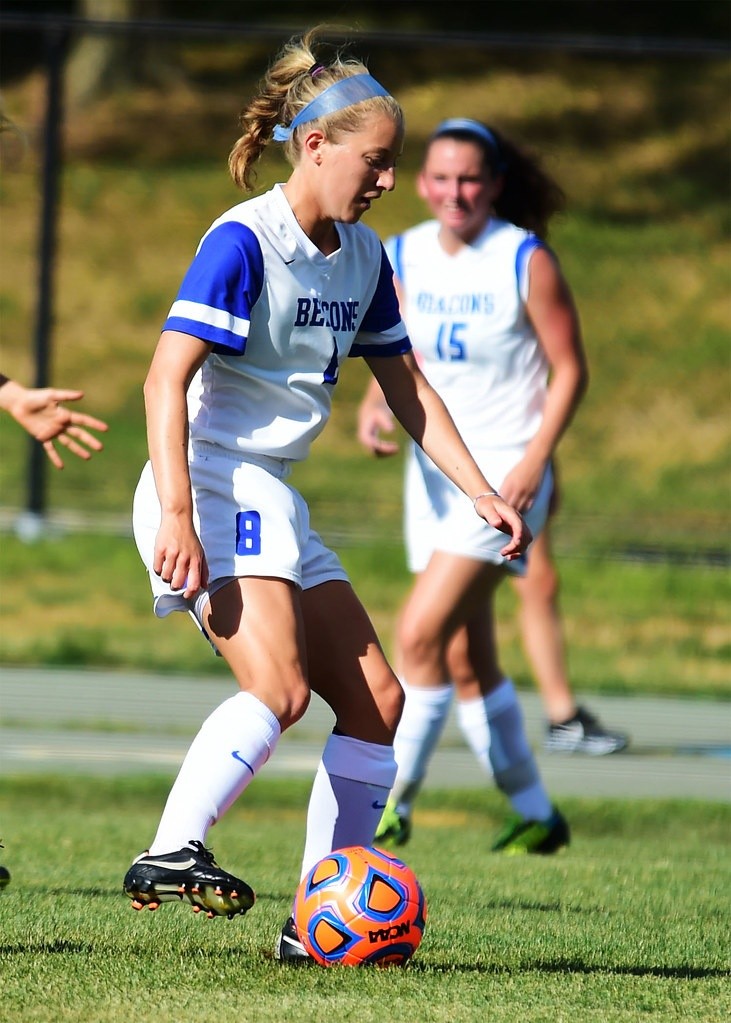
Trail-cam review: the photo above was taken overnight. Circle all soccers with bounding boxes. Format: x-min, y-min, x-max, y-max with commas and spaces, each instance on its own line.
293, 845, 429, 971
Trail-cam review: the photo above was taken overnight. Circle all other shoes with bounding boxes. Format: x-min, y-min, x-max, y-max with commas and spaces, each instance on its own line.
374, 796, 412, 847
0, 841, 11, 888
490, 806, 570, 855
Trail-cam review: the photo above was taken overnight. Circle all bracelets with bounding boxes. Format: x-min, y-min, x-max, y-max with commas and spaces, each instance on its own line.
473, 493, 497, 507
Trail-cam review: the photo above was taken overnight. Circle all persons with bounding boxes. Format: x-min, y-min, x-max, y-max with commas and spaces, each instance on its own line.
526, 457, 631, 754
358, 118, 588, 853
0, 371, 109, 889
124, 24, 532, 965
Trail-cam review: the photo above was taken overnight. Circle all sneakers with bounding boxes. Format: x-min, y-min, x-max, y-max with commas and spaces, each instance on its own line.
123, 840, 255, 920
545, 706, 629, 755
274, 916, 317, 966
